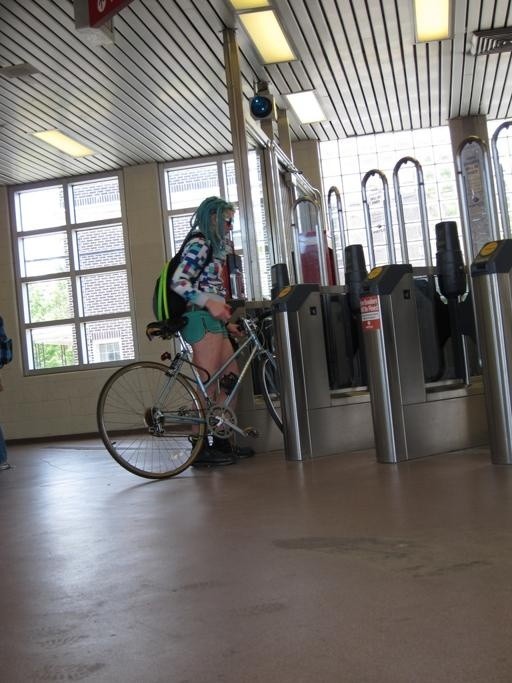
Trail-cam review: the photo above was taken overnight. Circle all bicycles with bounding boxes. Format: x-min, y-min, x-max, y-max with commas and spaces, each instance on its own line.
93, 302, 287, 484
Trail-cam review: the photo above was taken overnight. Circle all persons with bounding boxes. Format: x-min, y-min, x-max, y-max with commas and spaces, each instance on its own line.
0, 314, 12, 470
169, 196, 255, 467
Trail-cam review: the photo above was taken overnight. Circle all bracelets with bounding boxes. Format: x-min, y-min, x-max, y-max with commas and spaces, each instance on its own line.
224, 321, 229, 327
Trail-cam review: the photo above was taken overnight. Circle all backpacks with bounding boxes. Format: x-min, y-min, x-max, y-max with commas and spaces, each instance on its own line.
0, 315, 14, 370
152, 233, 213, 339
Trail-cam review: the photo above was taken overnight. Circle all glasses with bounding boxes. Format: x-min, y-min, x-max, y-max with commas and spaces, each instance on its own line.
223, 217, 233, 226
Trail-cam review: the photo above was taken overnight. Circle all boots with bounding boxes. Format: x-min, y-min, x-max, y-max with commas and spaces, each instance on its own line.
188, 433, 255, 468
